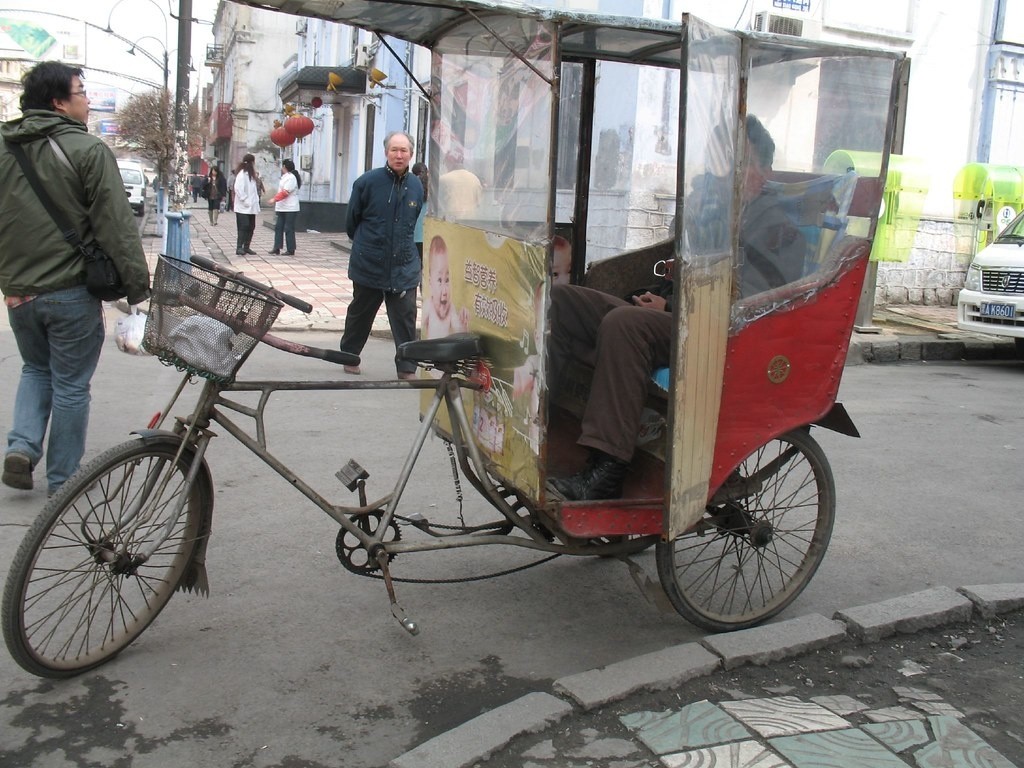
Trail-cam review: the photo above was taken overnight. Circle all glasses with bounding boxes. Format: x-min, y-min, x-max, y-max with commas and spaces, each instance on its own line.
69, 89, 87, 98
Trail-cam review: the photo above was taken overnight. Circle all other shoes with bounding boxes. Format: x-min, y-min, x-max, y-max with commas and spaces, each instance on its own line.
282, 249, 294, 255
1, 453, 33, 490
244, 248, 256, 255
344, 364, 360, 375
48, 465, 96, 499
236, 249, 245, 255
399, 371, 418, 380
269, 249, 280, 254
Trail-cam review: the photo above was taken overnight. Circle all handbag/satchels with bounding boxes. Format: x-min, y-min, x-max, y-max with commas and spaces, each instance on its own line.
201, 177, 213, 198
83, 245, 127, 301
116, 301, 156, 357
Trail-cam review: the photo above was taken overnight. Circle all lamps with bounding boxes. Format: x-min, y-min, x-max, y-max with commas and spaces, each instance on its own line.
367, 68, 388, 91
283, 104, 296, 117
325, 72, 344, 95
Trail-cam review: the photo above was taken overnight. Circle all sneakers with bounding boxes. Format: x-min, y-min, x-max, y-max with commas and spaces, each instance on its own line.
544, 457, 628, 502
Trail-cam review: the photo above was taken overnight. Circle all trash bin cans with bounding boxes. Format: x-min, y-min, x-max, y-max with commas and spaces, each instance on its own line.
164, 210, 192, 281
158, 187, 164, 210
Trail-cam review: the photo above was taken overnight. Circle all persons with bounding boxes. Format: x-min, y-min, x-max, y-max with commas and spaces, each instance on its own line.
438, 148, 484, 219
340, 131, 424, 379
152, 154, 301, 254
420, 235, 470, 340
412, 163, 428, 296
550, 116, 807, 502
2, 60, 152, 497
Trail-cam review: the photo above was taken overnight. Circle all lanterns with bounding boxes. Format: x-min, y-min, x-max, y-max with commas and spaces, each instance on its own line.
270, 105, 315, 151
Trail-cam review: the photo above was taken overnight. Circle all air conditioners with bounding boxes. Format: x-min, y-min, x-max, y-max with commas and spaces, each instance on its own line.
300, 154, 312, 170
295, 19, 306, 34
353, 43, 370, 67
753, 11, 808, 37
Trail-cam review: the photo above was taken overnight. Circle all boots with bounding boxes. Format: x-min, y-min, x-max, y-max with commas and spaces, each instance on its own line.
208, 210, 213, 226
212, 209, 218, 226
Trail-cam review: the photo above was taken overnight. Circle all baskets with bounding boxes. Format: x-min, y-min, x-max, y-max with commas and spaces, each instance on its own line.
142, 252, 283, 383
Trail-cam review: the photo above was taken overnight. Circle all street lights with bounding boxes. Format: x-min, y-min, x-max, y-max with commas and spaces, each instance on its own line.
105, 0, 197, 119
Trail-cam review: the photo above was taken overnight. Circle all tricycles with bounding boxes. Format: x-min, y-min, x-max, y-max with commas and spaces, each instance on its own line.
0, 0, 915, 682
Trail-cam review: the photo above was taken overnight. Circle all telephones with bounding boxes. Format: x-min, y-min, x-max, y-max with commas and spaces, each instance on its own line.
972, 198, 994, 232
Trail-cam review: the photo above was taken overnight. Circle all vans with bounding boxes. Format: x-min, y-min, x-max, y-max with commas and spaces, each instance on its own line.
957, 206, 1024, 354
115, 158, 148, 215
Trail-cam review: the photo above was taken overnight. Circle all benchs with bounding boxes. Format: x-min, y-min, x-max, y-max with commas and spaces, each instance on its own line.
588, 171, 882, 398
